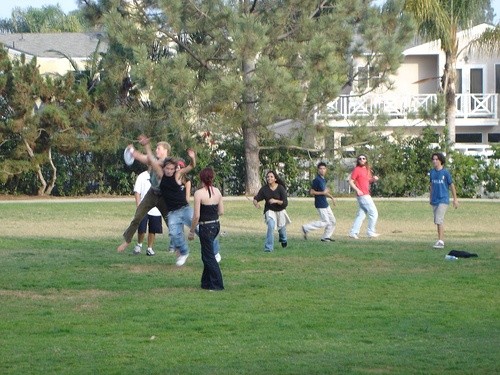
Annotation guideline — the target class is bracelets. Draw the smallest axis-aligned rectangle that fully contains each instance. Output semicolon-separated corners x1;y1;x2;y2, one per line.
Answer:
130;147;134;153
190;230;195;233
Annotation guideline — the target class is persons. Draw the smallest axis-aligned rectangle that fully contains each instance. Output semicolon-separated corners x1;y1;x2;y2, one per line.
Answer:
188;167;225;292
117;141;186;257
138;134;222;266
428;152;459;249
134;158;163;255
168;157;191;254
253;171;291;252
348;153;380;239
302;162;336;242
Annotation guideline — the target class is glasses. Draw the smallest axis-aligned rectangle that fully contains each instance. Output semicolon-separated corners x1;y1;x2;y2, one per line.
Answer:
358;158;365;160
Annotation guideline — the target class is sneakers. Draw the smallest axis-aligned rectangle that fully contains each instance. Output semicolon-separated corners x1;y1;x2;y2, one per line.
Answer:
176;253;189;266
215;253;221;263
281;242;287;247
303;226;307;240
133;243;142;252
349;234;358;239
433;241;444;248
146;247;155;255
320;238;335;242
368;232;379;237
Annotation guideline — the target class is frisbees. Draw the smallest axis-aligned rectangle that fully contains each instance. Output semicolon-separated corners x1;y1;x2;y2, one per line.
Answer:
124;145;135;165
